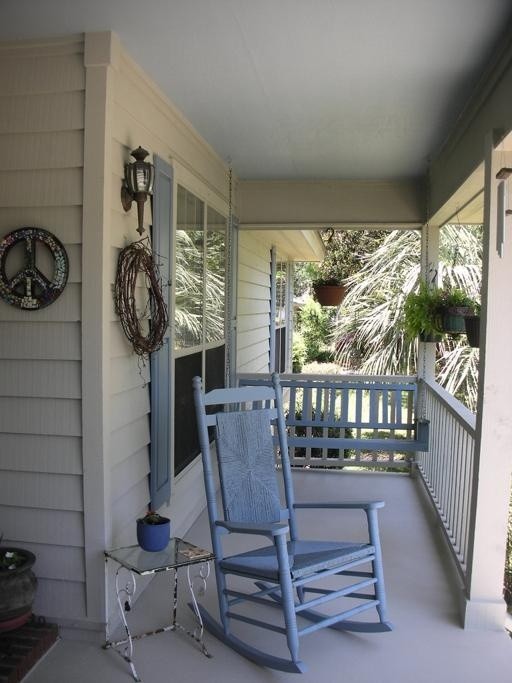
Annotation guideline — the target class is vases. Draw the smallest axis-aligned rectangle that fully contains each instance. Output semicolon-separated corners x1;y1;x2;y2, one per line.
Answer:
0;546;40;633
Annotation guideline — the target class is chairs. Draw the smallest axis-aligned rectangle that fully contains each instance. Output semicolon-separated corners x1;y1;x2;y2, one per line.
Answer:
190;370;393;676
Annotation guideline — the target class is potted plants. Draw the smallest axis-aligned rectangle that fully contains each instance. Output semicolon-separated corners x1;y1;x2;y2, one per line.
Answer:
311;270;347;307
402;286;481;350
137;511;171;552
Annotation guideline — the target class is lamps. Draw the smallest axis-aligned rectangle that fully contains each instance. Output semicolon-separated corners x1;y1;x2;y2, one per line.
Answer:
121;146;156;236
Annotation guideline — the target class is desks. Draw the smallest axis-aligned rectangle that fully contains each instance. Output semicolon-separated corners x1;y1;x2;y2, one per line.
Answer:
103;537;224;683
